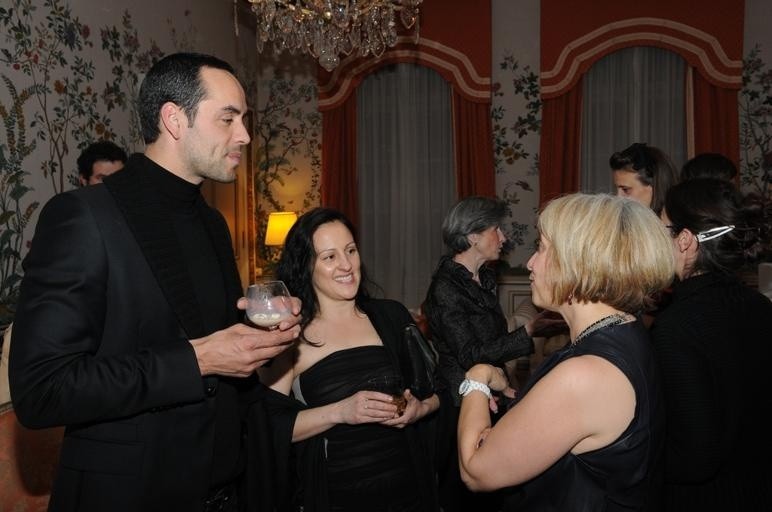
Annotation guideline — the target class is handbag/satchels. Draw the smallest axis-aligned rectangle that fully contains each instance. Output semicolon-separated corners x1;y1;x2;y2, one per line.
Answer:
400;325;437;397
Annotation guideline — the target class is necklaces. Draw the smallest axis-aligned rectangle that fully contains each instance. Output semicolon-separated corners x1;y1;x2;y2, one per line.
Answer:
570;314;623;349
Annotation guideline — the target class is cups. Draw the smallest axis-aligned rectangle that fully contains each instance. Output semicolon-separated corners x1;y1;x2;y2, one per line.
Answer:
368;375;406;414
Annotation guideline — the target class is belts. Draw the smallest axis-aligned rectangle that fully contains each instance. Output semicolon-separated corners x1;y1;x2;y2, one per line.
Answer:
204;483;238;512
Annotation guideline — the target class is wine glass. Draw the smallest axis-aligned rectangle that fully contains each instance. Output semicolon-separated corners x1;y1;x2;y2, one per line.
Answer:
243;282;294;345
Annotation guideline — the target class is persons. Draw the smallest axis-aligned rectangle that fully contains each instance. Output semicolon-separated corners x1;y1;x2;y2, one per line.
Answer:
8;50;304;512
260;206;451;512
420;195;541;512
77;140;128;187
651;177;772;512
680;152;738;184
609;142;678;218
455;193;676;512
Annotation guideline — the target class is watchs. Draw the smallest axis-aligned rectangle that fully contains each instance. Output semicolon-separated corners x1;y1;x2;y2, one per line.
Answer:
458;377;492;399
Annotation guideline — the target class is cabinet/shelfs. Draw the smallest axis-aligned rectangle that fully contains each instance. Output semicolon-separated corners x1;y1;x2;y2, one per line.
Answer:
493;273;758;405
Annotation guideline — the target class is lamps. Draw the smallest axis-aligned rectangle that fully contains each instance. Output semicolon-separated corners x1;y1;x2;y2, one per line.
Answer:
247;0;423;72
263;212;299;246
